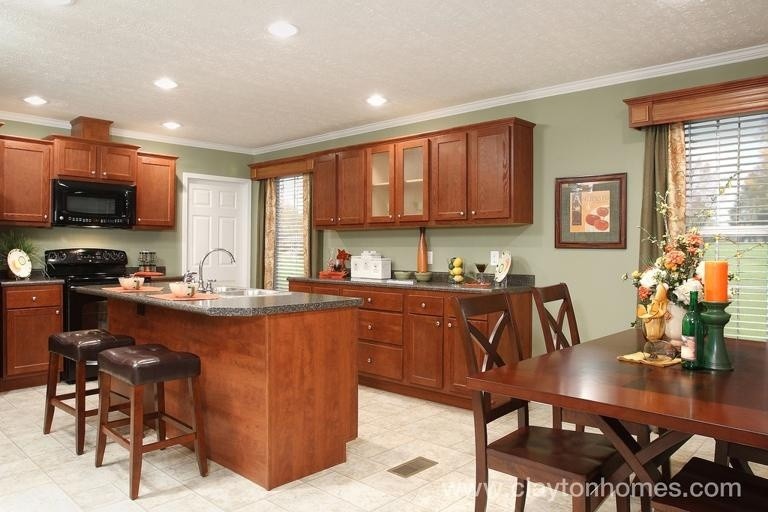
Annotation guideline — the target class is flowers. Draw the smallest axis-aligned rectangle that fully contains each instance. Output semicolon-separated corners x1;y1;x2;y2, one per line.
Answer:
621;171;768;305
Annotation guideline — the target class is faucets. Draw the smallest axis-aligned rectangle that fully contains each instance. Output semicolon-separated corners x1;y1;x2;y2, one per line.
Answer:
199;247;235;293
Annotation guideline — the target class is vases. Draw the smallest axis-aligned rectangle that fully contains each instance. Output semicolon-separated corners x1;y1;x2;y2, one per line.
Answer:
663;302;686;346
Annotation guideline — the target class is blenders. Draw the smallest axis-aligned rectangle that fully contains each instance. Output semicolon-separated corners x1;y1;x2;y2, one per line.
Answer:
137;251;157;272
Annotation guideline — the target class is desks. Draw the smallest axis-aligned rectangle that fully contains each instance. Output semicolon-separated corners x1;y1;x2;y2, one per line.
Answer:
465;328;767;512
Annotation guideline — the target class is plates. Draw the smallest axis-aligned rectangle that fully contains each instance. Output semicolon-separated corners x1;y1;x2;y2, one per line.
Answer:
494;253;512;283
7;248;32;278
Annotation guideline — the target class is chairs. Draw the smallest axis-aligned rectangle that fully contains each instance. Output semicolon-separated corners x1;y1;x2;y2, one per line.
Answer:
651;456;768;511
532;283;671;498
454;291;633;512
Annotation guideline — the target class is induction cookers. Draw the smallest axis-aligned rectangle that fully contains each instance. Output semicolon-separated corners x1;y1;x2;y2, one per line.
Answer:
44;248;130;285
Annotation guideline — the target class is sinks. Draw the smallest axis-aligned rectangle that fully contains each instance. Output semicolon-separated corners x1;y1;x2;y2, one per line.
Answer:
219;287;281;298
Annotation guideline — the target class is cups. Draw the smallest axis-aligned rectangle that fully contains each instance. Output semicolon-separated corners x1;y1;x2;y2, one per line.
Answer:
333;259;344;272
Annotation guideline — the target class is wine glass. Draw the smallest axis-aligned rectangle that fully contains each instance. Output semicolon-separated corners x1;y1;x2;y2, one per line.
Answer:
642;317;667;362
474;263;489;284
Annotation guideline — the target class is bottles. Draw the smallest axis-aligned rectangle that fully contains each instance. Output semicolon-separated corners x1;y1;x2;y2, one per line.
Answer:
680;290;705;371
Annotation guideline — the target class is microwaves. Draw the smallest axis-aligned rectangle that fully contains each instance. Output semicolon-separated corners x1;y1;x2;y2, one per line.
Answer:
52;179;136;229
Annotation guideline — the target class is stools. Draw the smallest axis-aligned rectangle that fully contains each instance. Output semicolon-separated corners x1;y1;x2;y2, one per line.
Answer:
43;328;135;454
96;345;209;500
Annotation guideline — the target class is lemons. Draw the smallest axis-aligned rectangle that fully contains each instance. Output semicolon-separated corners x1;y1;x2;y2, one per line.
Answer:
449;257;463;283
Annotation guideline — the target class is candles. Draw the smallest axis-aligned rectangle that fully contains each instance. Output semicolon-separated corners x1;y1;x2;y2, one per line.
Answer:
698;261;733;301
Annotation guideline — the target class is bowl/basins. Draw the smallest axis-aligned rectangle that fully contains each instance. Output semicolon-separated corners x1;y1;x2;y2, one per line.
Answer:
168;282;200;298
414;271;433;282
118;276;145;290
394;271;412;280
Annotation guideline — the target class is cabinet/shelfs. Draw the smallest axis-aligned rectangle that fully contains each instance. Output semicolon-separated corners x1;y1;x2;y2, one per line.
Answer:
312;117;536;231
52;135;139;182
137;152;179;229
1;284;64;392
0;136;54;227
290;281;532;412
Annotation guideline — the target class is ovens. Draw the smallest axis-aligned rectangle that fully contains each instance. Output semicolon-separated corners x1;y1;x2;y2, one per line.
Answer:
61;287;107;385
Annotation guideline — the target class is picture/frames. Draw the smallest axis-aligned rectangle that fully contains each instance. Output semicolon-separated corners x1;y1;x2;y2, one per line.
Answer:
555;173;626;249
